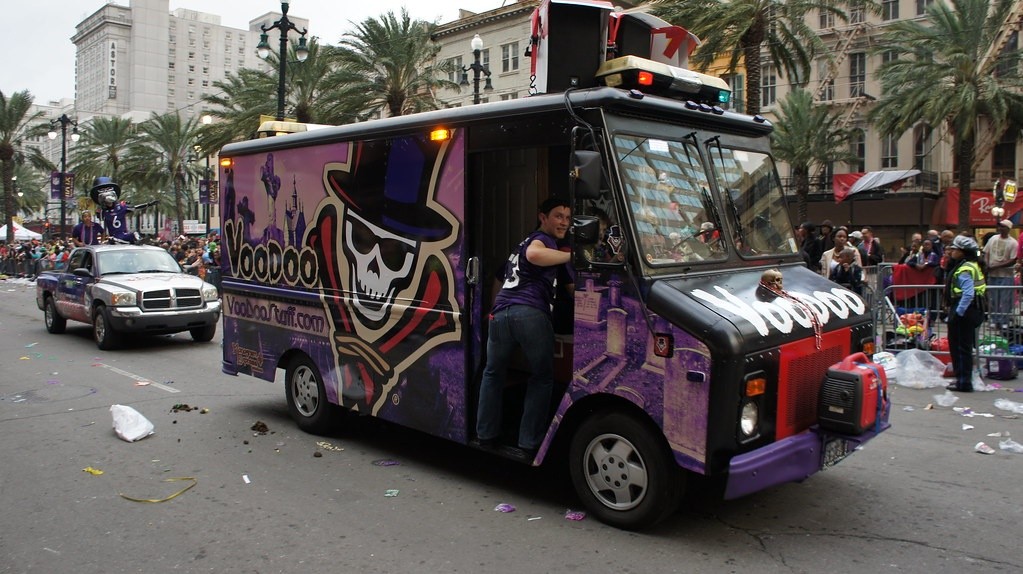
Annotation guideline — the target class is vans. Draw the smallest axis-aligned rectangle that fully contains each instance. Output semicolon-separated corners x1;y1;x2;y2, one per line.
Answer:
217;55;893;531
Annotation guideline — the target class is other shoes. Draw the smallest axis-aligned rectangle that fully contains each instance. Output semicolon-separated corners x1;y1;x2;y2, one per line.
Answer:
944;316;949;322
950;380;957;385
989;323;996;328
1002;323;1008;329
478;438;498;447
929;320;934;326
519;448;536;458
946;384;972;391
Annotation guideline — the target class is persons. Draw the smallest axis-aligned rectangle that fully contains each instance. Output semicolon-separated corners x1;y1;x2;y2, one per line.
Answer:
71;211;106;248
982;218;1019;324
0;231;222;293
477;195;611;456
818;225;863;279
946;234;987;392
791;220;1023;327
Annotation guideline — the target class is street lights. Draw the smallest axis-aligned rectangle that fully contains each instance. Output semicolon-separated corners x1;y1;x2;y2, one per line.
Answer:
458;33;494;105
46;113;81;240
256;0;310;121
11;175;24;216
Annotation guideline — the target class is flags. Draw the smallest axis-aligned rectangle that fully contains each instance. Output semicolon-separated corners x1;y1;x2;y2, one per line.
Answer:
833;168;922;205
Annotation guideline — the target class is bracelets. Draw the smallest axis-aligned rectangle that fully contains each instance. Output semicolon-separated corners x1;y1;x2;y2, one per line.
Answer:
570;250;574;262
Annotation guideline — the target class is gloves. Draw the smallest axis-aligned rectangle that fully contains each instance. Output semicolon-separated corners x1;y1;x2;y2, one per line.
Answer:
948;312;961;327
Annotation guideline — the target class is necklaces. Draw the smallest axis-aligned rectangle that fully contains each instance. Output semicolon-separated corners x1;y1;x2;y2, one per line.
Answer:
81;221;92;245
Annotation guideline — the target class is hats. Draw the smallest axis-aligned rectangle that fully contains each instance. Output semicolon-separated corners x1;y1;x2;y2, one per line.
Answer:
535;197;570;232
848;231;864;239
1000;219;1013;229
815;220;833;232
950;236;979;253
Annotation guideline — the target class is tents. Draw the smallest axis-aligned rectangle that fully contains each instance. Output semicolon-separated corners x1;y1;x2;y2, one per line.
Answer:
0;219;44;240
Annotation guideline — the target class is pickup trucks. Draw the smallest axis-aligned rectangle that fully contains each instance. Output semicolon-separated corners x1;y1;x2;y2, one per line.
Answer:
36;242;222;349
